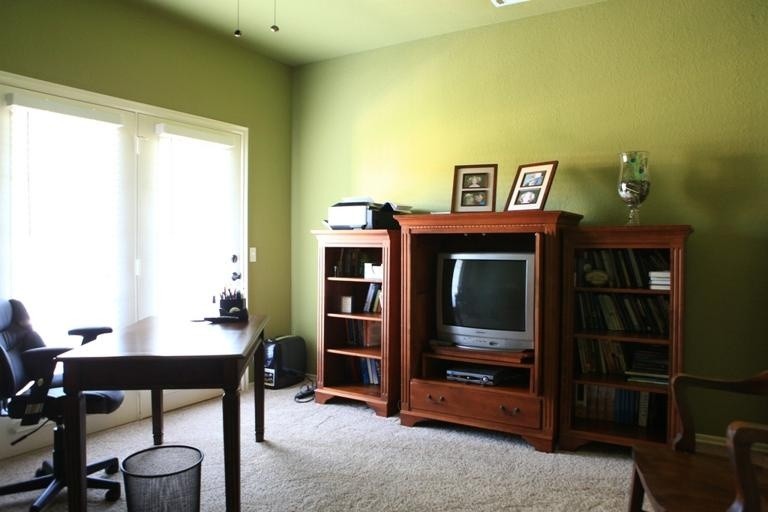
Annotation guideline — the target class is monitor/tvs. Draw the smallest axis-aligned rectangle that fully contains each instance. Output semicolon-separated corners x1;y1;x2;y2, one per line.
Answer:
436;252;535;353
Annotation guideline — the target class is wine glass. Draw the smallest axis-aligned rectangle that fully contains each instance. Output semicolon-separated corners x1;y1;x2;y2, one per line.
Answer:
615;151;650;224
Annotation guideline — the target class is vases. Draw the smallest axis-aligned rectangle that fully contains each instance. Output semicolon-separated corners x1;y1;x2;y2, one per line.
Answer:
612;148;657;227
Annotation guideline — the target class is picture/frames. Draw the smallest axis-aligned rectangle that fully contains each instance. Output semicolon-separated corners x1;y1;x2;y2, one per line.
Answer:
450;163;498;213
503;159;559;211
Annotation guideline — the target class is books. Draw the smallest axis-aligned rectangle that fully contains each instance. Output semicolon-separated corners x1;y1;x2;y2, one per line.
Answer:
330;248;383;384
571;244;672;431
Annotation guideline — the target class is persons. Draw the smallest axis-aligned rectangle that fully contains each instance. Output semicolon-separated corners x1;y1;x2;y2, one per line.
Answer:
463;174;487;206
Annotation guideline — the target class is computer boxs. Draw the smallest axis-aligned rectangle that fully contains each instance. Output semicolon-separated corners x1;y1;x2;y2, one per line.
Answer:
263;335;307;389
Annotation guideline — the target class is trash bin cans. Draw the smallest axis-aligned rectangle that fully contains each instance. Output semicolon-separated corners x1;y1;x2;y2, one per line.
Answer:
120;445;204;512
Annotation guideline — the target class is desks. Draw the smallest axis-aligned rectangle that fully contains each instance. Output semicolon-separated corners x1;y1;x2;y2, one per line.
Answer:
49;306;278;512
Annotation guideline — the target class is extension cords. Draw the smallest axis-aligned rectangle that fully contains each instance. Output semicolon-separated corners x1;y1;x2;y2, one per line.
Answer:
296;386;318;399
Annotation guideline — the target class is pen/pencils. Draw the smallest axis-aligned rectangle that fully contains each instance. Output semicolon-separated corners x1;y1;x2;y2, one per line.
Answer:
220;287;244;300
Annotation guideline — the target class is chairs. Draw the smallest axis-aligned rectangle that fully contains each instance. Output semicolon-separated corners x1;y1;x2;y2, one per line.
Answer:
0;291;124;512
619;361;768;511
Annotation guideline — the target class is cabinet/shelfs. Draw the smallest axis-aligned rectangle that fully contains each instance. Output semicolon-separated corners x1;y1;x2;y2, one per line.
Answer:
392;210;583;454
562;224;695;457
309;225;403;418
